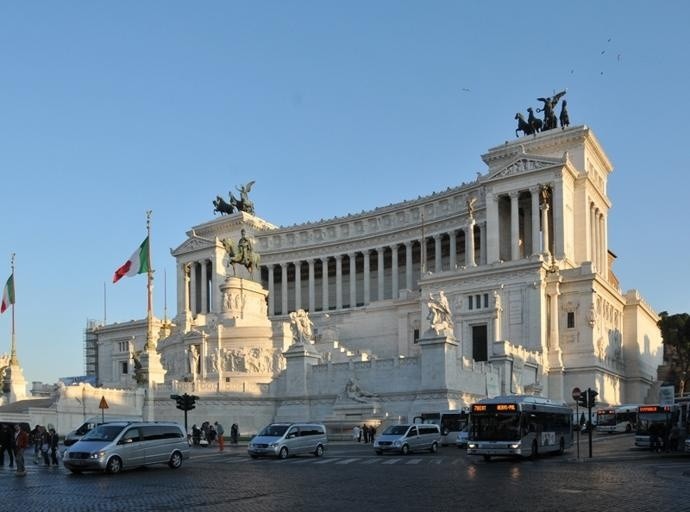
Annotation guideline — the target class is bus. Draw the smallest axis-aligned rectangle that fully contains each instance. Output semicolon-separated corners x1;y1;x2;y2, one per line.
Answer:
413;393;690;465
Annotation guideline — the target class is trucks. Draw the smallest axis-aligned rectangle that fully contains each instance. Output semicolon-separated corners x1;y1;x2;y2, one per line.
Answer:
62;414;144;446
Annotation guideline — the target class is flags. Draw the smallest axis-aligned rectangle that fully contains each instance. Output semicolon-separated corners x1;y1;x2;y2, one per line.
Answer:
0;274;15;314
112;236;150;283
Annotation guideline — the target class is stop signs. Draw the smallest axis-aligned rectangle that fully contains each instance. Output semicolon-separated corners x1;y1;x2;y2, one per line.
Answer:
571;387;582;401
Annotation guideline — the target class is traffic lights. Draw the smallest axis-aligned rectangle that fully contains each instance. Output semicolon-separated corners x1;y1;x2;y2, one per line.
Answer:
589;388;598;407
175;395;185;411
578;390;588;408
186;395;196;411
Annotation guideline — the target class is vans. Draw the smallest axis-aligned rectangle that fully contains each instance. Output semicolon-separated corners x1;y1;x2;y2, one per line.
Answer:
58;419;192;478
245;421;330;461
0;421;34;448
372;422;443;456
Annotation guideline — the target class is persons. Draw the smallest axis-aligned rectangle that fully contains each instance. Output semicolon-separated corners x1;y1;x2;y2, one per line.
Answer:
436;290;451;323
187;420;240;452
11;422;27;476
354;424;375;444
238;227;252;266
0;422;14;468
647;418;688;454
288;309;313;346
29;423;59;468
